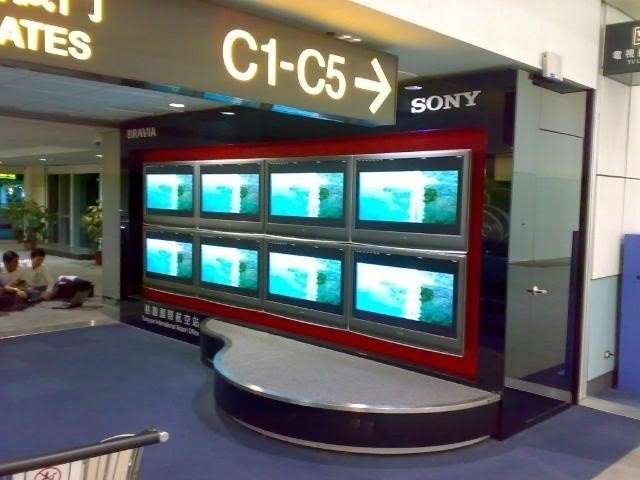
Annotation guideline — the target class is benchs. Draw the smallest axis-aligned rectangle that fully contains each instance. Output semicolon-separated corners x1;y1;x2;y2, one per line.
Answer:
200;315;500;457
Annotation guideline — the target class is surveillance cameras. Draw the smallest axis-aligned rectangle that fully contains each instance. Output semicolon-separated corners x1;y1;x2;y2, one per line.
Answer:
94;137;101;146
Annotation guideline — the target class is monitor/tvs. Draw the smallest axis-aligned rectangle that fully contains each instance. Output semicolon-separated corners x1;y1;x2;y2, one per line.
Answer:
264;234;351;329
201;159;263;231
354;150;473;253
264;154;355;242
198;231;264;310
352;246;467;357
140;161;197;227
144;228;198;294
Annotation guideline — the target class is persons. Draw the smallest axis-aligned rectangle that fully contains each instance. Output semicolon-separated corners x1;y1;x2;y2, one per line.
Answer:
19;248;53;302
1;250;35;312
